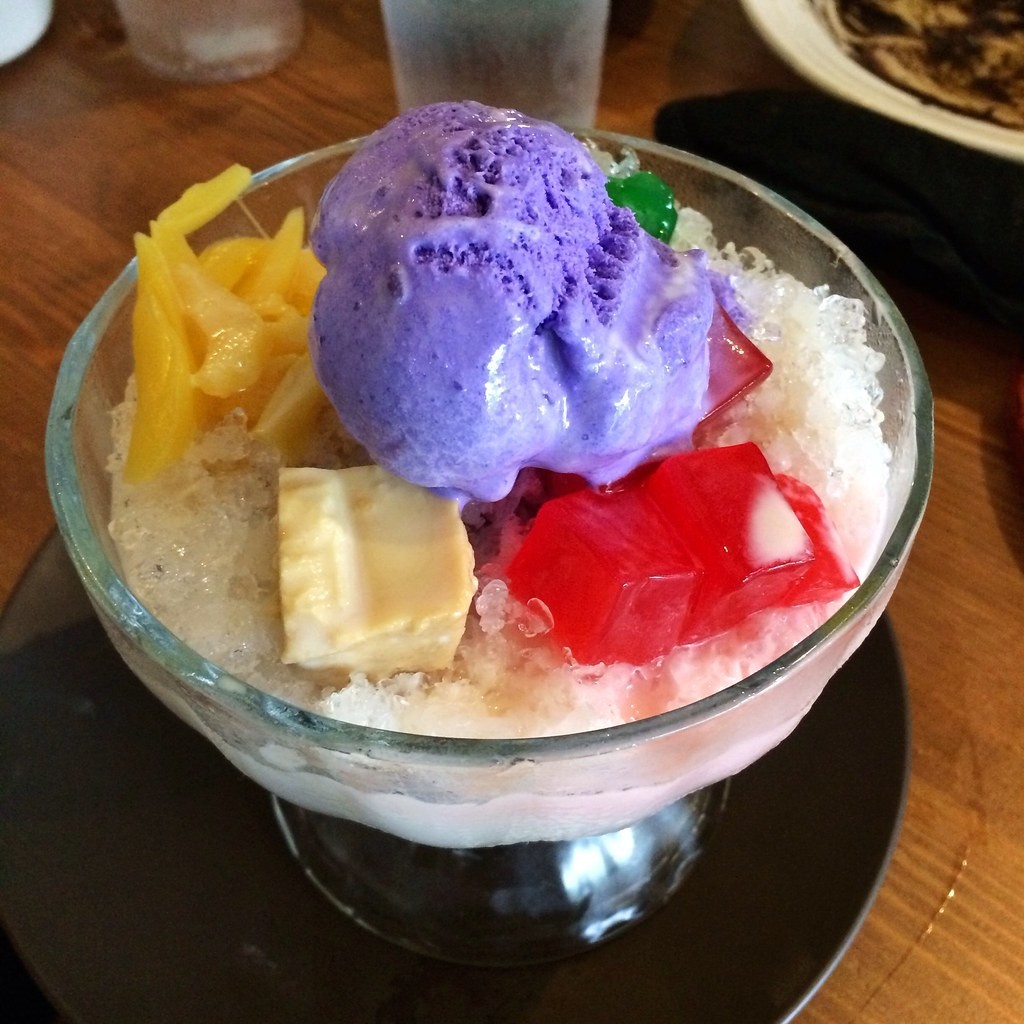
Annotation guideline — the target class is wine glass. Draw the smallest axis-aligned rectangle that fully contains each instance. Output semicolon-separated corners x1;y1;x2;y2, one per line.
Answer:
42;124;937;969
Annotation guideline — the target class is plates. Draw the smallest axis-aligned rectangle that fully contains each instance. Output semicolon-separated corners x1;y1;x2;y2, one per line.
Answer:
741;0;1024;164
0;524;910;1024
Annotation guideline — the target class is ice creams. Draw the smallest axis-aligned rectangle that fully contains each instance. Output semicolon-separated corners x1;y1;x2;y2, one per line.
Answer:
301;96;761;508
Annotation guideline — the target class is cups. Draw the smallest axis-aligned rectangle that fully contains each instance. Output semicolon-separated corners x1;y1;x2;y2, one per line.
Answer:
379;0;610;126
0;0;55;65
114;0;303;84
